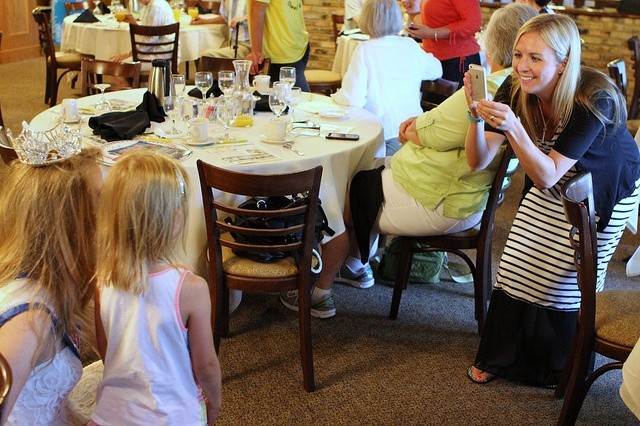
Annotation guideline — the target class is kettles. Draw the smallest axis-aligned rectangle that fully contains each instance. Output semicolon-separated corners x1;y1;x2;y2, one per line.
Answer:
147;58;176;113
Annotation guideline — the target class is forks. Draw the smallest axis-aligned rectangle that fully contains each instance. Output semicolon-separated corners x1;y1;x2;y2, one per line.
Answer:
284;144;305;157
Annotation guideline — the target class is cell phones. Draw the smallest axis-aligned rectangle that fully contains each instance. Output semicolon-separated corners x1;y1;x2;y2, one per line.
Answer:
409;27;417;30
469;64;488;102
326;133;359;140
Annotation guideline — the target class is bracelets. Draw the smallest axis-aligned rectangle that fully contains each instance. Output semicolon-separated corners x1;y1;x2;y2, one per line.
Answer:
436;27;438;41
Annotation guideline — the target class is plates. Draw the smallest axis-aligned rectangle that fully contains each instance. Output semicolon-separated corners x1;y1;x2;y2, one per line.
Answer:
93;139;190;167
260;134;295;143
300;100;349;118
185;138;215;146
350;33;371;41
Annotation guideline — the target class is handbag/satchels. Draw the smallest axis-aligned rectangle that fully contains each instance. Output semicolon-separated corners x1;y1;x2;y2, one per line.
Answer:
224;192;335;274
378;237;474;283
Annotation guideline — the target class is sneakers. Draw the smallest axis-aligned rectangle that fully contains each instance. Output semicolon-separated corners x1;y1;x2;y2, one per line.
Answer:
333;263;375;288
280;290;336;318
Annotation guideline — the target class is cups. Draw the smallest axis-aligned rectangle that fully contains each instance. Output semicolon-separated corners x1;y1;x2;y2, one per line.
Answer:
189;117;210;143
264;119;288;141
253;75;269;95
62;98;80;119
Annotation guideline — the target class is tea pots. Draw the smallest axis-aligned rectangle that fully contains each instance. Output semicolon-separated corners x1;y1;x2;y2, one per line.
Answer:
233;58;254;121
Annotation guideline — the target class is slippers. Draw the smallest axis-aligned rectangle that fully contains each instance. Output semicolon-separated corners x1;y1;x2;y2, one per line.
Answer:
467;365;496;384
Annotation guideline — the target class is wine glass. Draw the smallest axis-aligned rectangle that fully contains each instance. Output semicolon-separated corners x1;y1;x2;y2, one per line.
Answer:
188;7;198;24
94;84;111;117
195;71;235;143
172;8;181;23
115;5;124;25
269;67;301;120
166;74;194;136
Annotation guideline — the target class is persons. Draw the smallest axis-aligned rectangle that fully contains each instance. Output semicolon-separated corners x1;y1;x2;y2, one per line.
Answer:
463;12;635;394
85;152;222;426
338;0;368;38
405;0;483;84
51;0;85;52
330;0;444;153
246;0;312;93
191;0;251;59
108;0;177;74
2;148;100;426
278;4;538;318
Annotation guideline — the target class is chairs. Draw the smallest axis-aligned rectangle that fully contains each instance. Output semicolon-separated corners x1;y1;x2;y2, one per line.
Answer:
558;170;640;425
31;7;103;107
420;78;460;112
67;2;89;16
0;108;19;165
626;35;640;120
128;22;180;83
80;57;140;98
303;13;345;97
198;1;219;14
197;159;324;392
199;55;271;85
607;57;640;137
381;140;512;336
